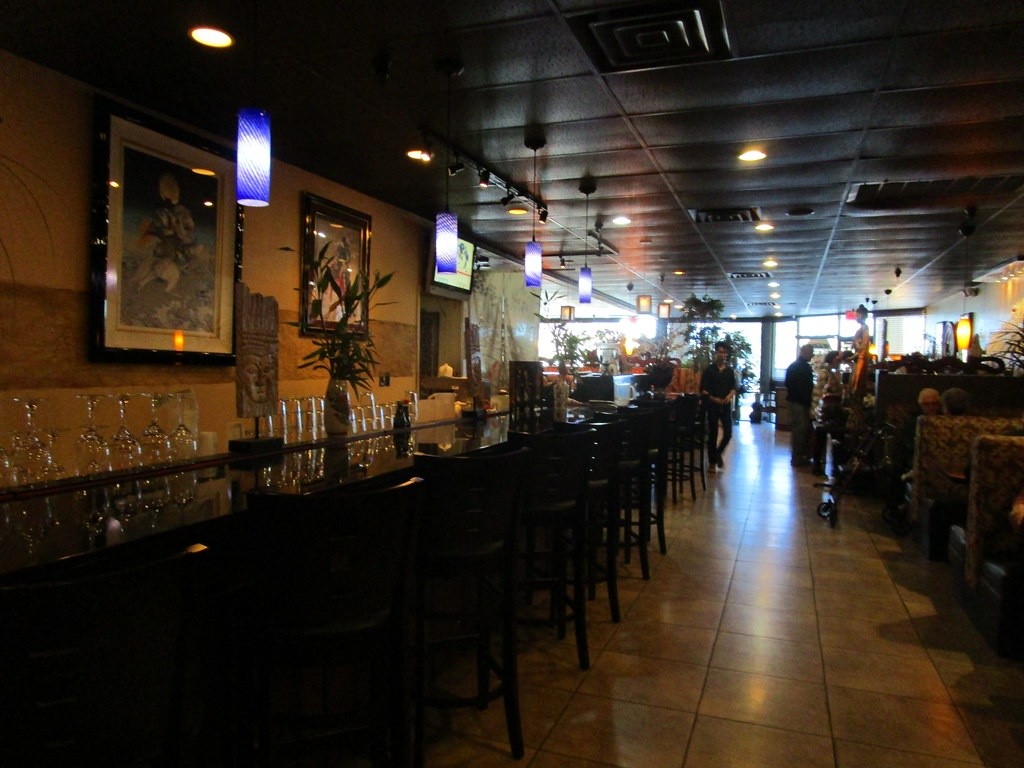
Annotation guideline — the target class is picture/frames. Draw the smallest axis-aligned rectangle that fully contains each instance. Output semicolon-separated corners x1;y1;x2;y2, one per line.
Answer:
84;92;238;364
297;189;368;338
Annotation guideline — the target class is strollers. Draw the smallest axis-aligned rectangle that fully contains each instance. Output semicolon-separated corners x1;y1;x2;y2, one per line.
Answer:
811;392;908;524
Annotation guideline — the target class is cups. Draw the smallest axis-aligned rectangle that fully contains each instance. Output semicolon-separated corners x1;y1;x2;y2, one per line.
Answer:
224;391;464;448
242;430;417;494
199;432;218;455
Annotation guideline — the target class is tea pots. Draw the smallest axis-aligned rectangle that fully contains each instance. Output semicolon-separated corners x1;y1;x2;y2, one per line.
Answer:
439;363;453;377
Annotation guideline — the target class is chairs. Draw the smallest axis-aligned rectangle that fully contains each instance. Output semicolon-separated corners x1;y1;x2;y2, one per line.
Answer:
240;390;712;768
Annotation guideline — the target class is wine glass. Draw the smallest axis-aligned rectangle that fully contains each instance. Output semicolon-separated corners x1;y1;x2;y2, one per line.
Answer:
0;467;198;567
0;394;80;491
166;390;198;466
107;393;142;476
75;394;112;480
140;392;176;471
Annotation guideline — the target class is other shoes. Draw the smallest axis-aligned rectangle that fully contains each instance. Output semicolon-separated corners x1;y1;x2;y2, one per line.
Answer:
716;449;724;468
708;463;716;473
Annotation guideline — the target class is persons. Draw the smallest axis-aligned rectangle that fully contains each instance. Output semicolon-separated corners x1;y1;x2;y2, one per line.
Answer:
894;350;965;374
889;388;972;517
964;422;1024;477
844;306;870;427
785;344;814;466
1009;489;1024;533
811;352;842;477
701;342;736;472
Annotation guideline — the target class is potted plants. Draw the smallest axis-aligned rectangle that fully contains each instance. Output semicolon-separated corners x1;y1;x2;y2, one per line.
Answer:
528;286;592;422
289;239;396;433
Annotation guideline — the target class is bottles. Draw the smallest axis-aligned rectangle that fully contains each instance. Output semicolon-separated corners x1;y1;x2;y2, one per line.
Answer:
393;434;403;458
600;362;614;401
554;373;569;422
167;378;200;445
402;432;410;457
401;400;411;427
393;401;409;428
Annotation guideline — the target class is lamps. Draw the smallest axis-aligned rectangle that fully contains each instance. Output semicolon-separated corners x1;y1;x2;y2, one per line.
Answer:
499;183;514;206
476;164;488;188
956;280;971;351
235;0;272;207
524;126;547;285
436;63;464;272
578;172;597;303
536;204;548;224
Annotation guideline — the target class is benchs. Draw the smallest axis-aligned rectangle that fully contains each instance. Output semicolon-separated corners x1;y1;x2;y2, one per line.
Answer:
880;399;1023;593
